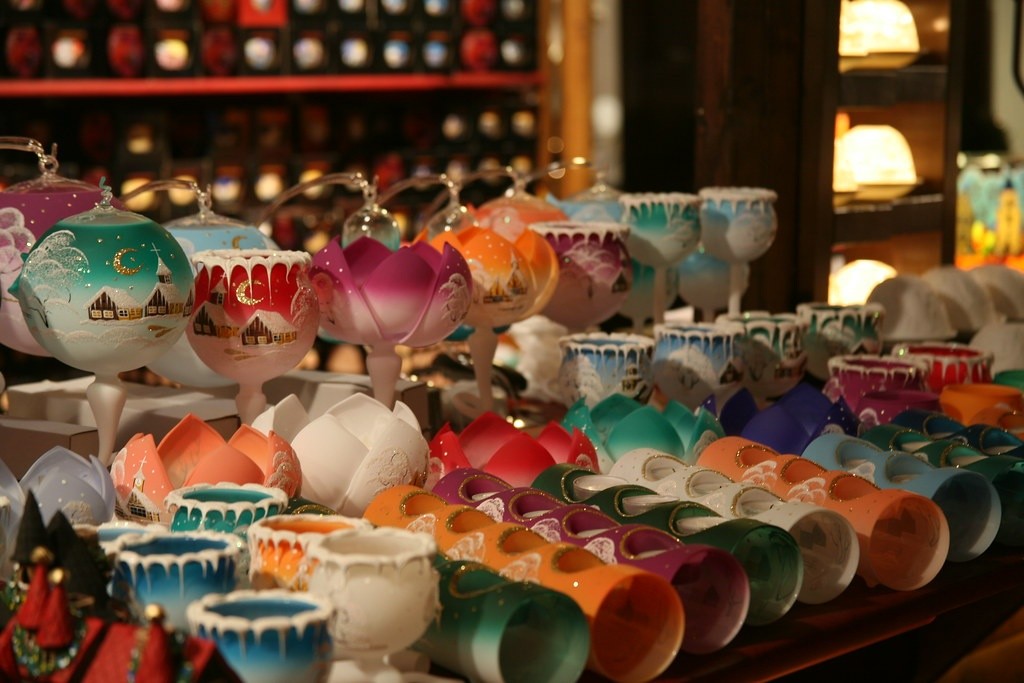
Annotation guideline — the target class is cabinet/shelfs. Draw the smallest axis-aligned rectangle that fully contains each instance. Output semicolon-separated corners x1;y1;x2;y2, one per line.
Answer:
2;1;622;220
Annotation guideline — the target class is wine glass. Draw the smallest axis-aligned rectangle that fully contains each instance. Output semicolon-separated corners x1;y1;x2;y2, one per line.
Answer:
522;189;995;418
194;248;318;428
82;483;440;682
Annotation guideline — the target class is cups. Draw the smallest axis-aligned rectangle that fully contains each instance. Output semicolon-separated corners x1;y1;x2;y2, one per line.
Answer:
360;368;1024;681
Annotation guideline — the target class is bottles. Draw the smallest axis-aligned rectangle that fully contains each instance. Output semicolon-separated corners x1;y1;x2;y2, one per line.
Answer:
5;104;537;243
2;2;523;77
